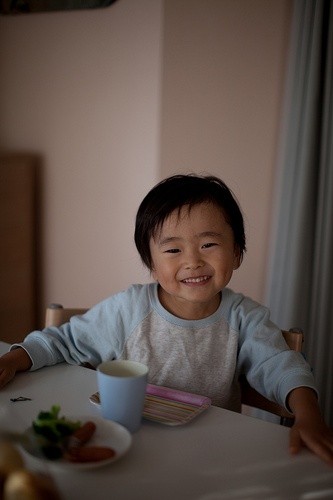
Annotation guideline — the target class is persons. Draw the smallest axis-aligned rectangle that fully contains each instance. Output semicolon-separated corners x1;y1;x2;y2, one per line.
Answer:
0;173;333;470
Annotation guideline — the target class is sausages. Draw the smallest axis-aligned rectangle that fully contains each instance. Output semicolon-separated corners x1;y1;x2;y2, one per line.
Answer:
71;422;96;444
67;446;117;460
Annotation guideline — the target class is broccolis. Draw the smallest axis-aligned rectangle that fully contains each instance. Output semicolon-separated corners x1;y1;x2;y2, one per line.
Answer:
24;405;79;460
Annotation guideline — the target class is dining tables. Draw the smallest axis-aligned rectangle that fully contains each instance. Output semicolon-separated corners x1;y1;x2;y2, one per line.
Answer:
0;341;332;500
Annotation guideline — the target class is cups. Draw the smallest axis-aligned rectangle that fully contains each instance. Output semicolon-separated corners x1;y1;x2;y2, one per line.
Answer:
97;358;148;435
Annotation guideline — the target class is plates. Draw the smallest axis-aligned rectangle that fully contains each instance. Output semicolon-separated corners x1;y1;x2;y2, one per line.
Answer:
89;382;212;427
19;414;135;472
